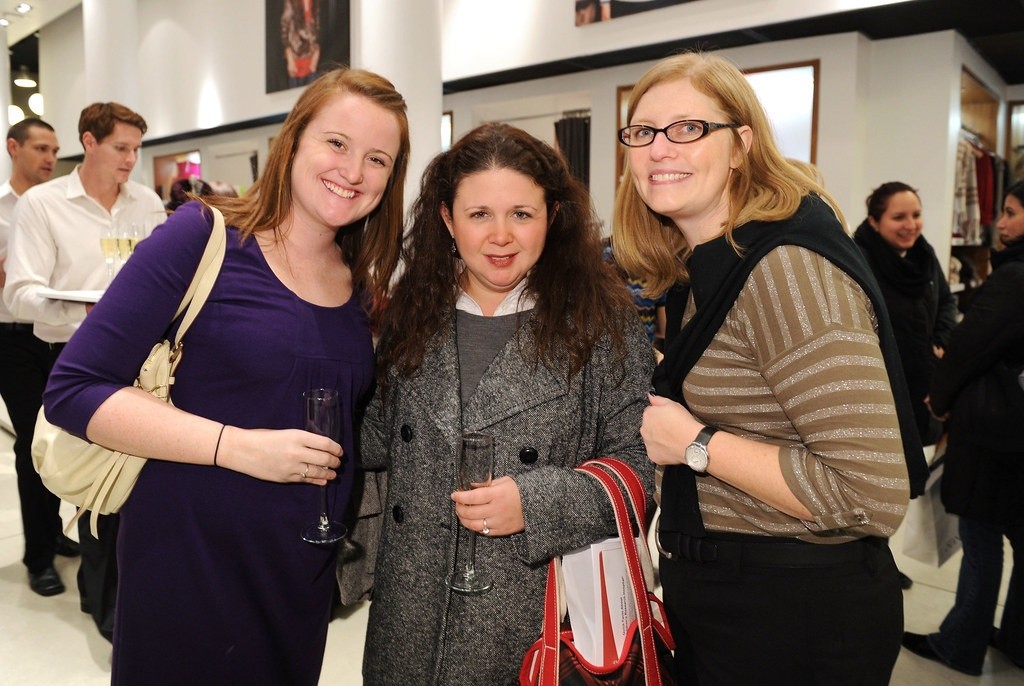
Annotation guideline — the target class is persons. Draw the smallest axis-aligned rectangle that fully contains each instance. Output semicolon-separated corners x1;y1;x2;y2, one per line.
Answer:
1;102;238;646
44;67;411;686
848;179;1024;676
361;120;656;686
604;244;668;349
612;51;931;686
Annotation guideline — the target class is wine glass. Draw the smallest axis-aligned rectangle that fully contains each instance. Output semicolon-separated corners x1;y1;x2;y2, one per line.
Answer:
444;431;493;592
302;386;350;544
96;220;145;297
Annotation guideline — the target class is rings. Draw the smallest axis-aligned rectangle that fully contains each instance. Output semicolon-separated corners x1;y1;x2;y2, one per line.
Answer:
481;519;489;533
303;462;310;478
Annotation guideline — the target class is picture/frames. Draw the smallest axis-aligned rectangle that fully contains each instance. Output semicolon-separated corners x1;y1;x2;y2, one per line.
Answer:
615;59;821;198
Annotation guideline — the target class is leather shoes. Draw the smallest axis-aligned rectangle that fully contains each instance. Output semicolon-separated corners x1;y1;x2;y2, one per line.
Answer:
31;568;64;596
59;536;80;557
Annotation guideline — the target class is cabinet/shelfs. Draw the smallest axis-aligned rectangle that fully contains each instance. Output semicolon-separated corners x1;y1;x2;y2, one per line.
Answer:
946;71;1024;297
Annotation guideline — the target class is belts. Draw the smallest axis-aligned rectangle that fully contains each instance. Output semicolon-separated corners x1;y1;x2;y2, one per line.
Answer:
0;323;33;330
655;516;888;564
32;334;66;351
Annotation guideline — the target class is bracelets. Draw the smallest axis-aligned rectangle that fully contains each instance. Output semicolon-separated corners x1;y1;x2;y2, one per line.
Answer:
214;425;226;466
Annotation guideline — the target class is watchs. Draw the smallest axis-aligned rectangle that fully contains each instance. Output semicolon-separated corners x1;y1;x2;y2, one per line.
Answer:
686;424;721;476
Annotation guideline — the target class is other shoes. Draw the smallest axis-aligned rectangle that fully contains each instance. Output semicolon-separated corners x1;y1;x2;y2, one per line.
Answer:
902;631;940;661
989;626;1003;650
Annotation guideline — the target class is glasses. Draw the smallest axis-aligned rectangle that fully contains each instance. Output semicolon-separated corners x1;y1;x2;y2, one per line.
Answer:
618;120;739;147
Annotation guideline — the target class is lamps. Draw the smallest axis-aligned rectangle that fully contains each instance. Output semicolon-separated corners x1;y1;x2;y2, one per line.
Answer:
15;64;36;88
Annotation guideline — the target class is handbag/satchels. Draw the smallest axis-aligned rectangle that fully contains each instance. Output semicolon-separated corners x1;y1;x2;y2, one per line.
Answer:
900;422;963;568
519;458;678;686
31;205;226;540
